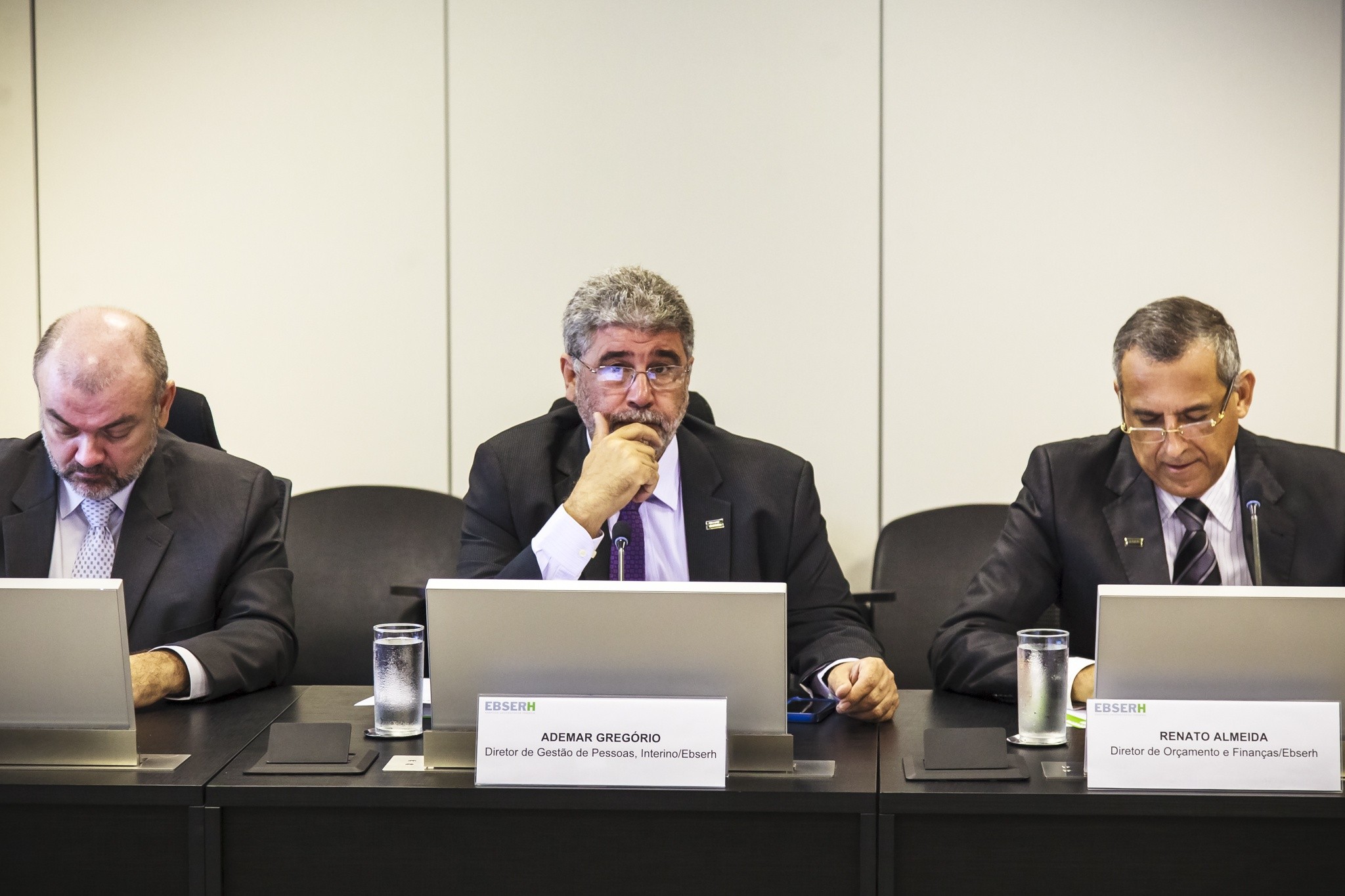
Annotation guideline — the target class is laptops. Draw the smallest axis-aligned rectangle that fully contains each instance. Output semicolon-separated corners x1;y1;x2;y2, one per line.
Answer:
0;578;135;731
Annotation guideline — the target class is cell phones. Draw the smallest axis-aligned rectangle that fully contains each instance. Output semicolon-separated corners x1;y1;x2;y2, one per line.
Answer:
787;697;836;724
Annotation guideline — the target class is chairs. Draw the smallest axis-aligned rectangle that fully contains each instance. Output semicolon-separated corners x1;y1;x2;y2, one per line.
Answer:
283;484;463;686
872;503;1018;688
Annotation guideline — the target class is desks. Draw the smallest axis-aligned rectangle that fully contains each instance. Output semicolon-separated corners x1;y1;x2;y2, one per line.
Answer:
0;690;1345;895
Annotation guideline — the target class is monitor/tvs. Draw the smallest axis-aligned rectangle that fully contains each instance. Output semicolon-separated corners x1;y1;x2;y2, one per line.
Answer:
425;578;788;737
1094;585;1345;740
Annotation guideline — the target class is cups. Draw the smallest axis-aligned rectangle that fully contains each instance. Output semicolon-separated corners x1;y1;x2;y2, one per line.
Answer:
373;623;424;737
1017;628;1069;743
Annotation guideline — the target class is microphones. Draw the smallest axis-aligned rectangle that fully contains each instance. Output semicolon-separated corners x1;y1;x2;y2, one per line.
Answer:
1241;479;1263;586
612;521;631;580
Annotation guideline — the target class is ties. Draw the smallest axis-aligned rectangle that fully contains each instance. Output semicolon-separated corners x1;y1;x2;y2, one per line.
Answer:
1174;498;1222;586
609;500;645;582
70;497;117;578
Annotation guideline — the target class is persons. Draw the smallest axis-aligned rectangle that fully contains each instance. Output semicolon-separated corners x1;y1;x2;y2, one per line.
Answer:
0;307;298;710
457;268;901;723
926;296;1345;710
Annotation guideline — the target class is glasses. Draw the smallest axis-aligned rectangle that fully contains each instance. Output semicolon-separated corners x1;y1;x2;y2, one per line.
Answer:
569;351;690;392
1120;372;1236;443
